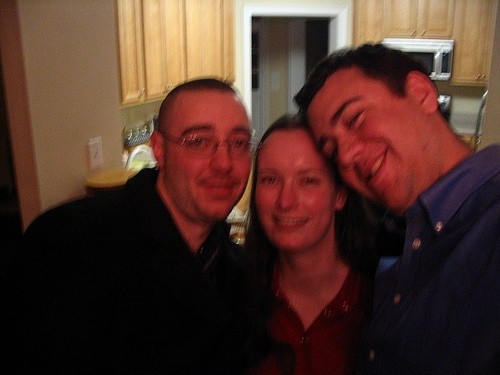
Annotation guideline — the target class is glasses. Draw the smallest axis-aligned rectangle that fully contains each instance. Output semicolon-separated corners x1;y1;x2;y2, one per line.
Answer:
168;129;262;162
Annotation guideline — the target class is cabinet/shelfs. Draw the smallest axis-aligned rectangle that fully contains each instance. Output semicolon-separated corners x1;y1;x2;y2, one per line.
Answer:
352;0;498;88
116;0;236;106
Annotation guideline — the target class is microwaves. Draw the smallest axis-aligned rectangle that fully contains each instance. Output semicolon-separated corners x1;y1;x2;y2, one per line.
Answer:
380;38;455;82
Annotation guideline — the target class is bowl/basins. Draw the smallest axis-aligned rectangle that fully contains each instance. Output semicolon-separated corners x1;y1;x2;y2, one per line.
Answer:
126;145;157;172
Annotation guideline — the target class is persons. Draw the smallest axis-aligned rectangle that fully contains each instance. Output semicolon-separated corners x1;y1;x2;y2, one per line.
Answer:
0;78;263;375
243;114;366;375
292;42;500;375
